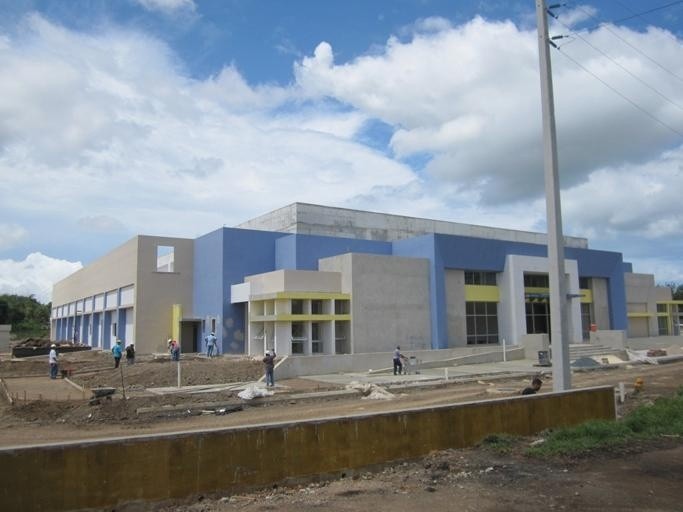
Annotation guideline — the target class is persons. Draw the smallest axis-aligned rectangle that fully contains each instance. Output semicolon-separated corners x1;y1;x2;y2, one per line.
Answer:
170;339;180;361
519;377;542;396
390;345;405;376
261;348;276;388
166;339;172;353
111;339;123;369
204;332;217;357
46;342;60;380
124;343;135;366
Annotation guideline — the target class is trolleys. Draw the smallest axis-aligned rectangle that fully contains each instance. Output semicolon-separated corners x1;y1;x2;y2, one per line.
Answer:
403;357;423;375
89;387;116;401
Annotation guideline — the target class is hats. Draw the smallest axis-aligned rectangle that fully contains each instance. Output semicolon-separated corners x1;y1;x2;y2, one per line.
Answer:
117;340;122;343
51;344;57;348
168;339;171;343
265;350;270;355
